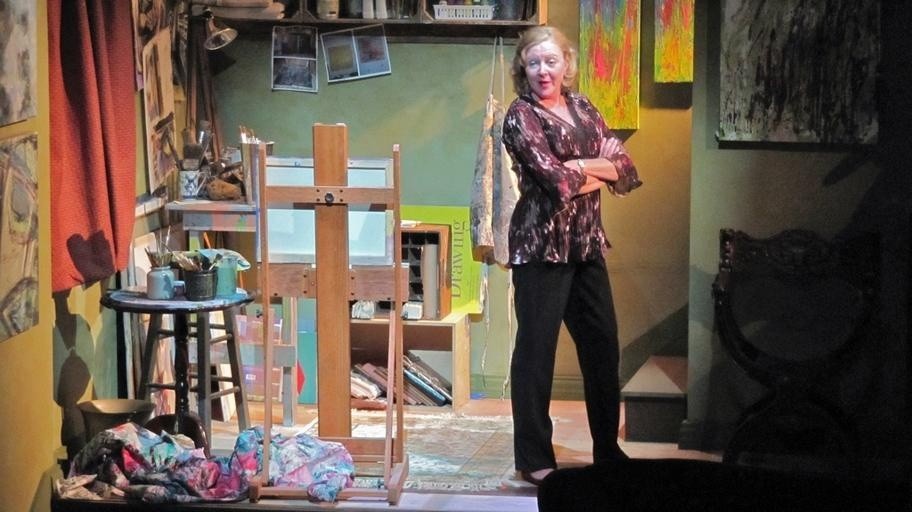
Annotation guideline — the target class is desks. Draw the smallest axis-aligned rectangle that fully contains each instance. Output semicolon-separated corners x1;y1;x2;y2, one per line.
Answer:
167;198;259;264
103;287;253;456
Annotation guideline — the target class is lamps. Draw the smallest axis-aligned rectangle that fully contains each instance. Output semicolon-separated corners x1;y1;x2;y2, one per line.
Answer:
200;5;240;54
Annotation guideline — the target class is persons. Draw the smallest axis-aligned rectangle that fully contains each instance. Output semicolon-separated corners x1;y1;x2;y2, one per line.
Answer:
497;25;643;483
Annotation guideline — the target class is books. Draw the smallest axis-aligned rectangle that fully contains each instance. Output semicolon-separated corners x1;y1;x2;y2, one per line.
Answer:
351;353;452;407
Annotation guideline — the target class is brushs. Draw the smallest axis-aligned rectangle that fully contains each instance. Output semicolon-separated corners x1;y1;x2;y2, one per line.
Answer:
183;144;202;170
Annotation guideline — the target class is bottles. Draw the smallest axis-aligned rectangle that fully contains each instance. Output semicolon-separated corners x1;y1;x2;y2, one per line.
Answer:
146;265;177;300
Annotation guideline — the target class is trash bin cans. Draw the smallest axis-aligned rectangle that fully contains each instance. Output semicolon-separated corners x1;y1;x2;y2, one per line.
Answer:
82;400;157;449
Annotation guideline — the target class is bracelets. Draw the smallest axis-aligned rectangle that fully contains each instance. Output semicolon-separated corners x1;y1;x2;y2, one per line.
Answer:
577;158;587;171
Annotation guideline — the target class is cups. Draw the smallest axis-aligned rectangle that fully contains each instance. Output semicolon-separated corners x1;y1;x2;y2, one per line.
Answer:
181;267;218;302
178;170;207;201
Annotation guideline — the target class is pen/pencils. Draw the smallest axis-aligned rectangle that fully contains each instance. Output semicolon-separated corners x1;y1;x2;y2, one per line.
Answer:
144;226;173;267
176;251;223;272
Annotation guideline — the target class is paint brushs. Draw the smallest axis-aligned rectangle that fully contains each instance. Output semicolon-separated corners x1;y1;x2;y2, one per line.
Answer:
181;120;214;169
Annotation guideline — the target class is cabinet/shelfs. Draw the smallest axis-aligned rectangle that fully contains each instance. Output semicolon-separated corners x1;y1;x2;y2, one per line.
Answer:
343;315;471;416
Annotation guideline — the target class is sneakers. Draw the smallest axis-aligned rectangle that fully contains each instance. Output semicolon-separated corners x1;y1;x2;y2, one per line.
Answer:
519;466;558;485
